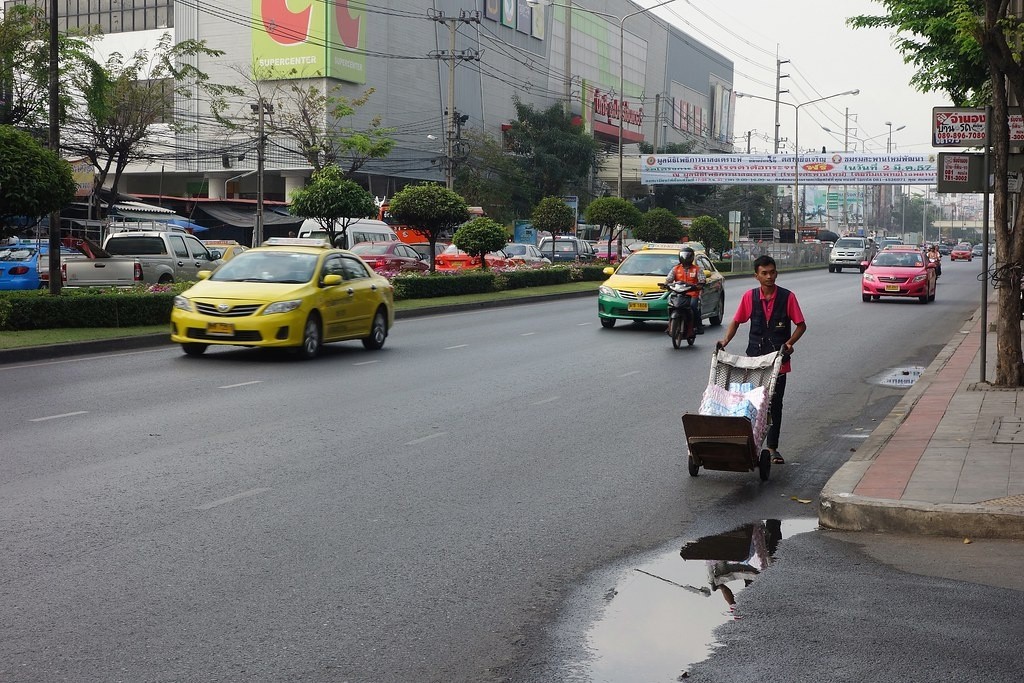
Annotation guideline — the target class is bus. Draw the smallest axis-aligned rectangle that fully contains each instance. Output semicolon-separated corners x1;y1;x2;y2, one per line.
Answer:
378;205;485;243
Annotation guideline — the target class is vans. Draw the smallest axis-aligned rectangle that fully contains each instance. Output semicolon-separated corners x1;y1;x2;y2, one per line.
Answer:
297;216;400;251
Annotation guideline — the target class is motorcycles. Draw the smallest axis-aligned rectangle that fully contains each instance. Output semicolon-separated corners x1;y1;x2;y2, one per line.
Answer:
657;280;700;349
928;256;943;281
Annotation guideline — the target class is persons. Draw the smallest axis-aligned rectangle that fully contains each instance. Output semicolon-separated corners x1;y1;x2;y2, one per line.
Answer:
716;256;806;464
927;245;943;275
662;248;706;333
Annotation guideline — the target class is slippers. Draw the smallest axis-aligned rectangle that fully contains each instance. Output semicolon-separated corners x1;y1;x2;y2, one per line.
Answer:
770;451;784;464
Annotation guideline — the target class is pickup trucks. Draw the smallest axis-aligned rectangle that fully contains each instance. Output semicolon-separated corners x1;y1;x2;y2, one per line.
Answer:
36;215;190;287
61;230;224;288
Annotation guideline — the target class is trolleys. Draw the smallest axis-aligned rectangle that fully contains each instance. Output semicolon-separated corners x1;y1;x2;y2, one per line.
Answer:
681;341;788;481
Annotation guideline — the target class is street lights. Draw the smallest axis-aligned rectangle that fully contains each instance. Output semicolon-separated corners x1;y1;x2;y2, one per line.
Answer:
736;89;907;241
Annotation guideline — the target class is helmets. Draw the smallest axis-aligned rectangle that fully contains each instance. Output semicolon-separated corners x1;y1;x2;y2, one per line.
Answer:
679;248;694;266
929;245;934;250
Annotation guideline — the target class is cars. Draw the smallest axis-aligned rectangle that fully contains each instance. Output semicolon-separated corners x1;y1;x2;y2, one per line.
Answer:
409;243;450;260
860;250;936;305
199;240;251;264
350;242;429;272
828;235;876;274
170;238;395;360
598;242;725;335
501;236;638;270
435;244;518;269
0;243;81;290
941;241;996;262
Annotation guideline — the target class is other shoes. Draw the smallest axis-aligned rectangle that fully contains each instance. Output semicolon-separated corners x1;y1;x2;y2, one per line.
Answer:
664;329;669;332
697;322;704;334
